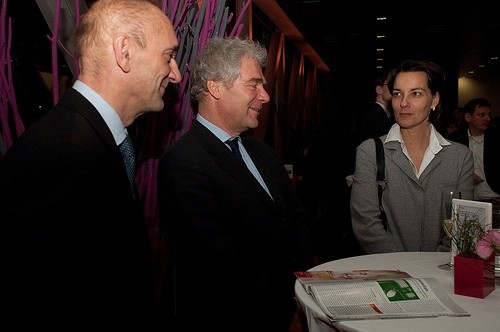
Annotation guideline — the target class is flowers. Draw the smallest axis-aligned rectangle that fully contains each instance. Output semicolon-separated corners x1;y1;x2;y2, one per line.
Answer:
439;201;500;259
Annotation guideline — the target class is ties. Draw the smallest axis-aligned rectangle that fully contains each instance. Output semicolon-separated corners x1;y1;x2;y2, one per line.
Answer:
118;133;135;202
226;137;253;177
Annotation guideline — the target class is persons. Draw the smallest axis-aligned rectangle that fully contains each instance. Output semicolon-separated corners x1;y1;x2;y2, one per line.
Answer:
0;0;182;332
294;270;411;281
158;37;310;332
350;60;500;254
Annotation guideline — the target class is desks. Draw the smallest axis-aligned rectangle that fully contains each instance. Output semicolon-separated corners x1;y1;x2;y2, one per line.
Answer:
295;251;500;332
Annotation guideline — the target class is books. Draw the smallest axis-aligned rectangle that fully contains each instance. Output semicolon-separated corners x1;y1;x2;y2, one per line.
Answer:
294;270;471;323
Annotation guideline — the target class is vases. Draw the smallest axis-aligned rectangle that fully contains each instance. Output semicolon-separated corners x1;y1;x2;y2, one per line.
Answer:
453;255;495;299
